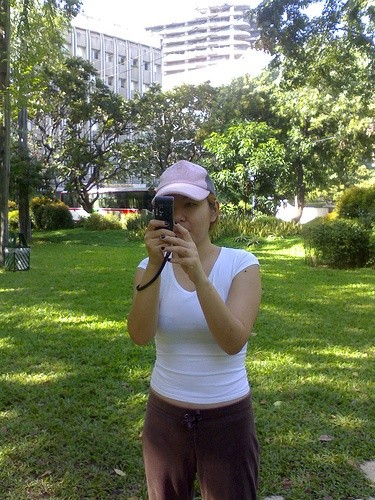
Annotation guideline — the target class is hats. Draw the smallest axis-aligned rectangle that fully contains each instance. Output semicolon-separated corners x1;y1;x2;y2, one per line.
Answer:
151;160;216;205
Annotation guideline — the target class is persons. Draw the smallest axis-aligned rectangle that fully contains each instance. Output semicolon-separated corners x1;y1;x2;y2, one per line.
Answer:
127;160;261;500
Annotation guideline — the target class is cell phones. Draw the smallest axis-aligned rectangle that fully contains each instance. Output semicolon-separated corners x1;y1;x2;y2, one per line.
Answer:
154;196;175;232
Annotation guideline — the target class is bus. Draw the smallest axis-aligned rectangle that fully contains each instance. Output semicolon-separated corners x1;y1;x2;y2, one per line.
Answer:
59;183;155;230
